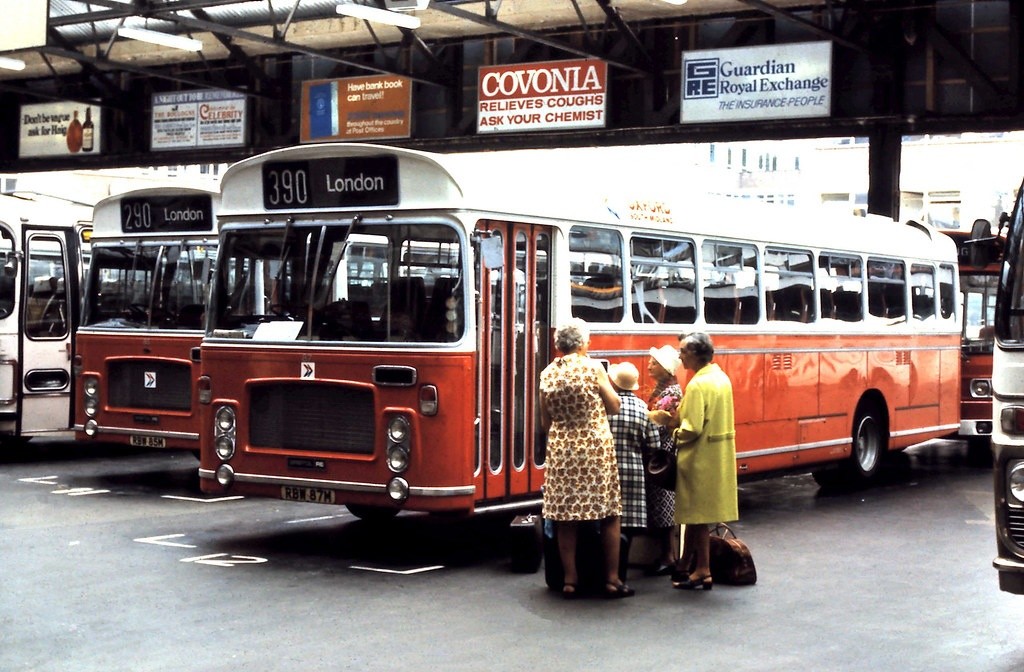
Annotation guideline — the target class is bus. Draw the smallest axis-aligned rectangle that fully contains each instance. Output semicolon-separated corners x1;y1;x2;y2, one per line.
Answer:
71;186;548;463
955;176;1024;599
0;189;94;447
195;143;963;549
937;226;1007;442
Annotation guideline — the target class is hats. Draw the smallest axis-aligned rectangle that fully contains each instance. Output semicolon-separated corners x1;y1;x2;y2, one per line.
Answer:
607;362;639;390
649;344;681;376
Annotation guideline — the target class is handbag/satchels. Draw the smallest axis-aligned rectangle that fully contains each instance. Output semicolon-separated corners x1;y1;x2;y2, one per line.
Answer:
687;522;756;586
642;430;679;491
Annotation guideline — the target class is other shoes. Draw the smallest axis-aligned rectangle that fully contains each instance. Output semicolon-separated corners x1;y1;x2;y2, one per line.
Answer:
644;559;676;577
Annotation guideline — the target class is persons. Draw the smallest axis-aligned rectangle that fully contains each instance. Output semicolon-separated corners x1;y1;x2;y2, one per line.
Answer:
538;317;740;598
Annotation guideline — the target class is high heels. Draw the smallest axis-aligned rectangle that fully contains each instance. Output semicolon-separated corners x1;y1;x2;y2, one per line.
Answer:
605;581;635;600
562;580;578;598
670;570;690;582
672;575;714;589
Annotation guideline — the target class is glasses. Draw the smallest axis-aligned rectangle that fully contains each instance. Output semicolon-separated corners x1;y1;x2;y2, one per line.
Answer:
586;340;591;346
